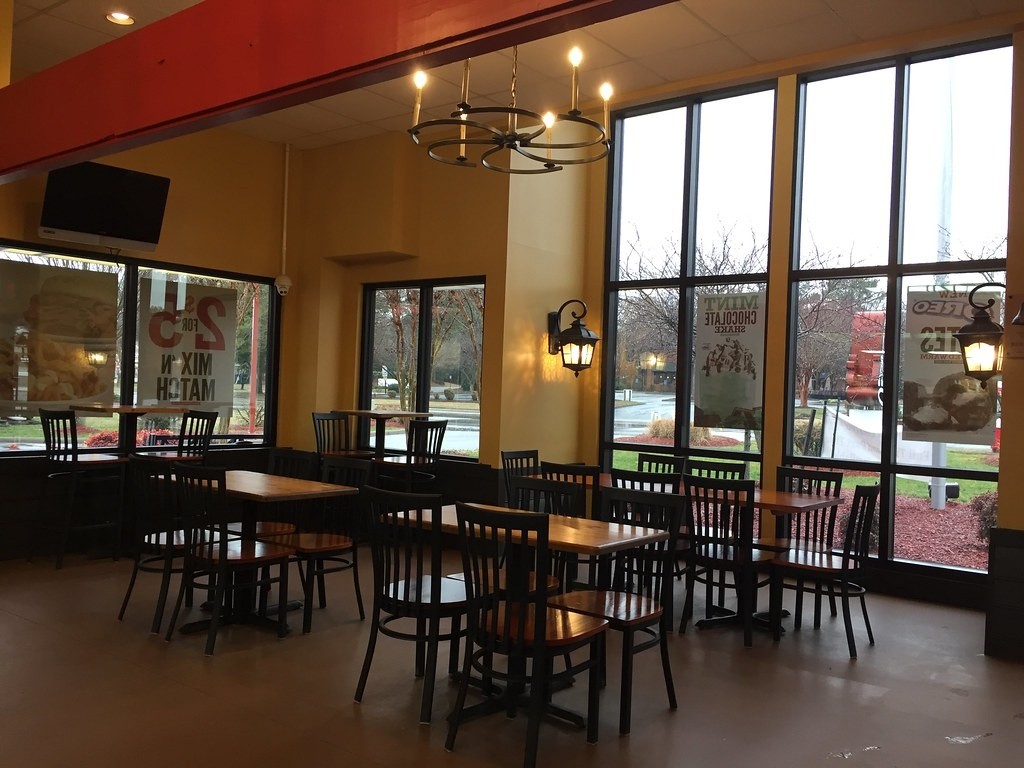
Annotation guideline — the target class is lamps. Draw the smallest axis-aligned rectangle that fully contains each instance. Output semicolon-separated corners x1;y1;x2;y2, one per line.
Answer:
949;281;1024;390
81;327;110;368
406;44;614;177
548;299;603;378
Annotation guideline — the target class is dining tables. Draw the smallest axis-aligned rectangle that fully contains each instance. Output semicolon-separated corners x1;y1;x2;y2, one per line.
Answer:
153;468;360;639
522;472;845;641
377;505;669;729
332;408;433;459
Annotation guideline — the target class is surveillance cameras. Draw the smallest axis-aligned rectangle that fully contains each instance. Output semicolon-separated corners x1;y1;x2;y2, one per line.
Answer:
274;275;293;297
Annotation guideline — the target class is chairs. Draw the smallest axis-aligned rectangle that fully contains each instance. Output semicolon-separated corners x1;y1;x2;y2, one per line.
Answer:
685;458;747;544
498;450;539;570
540;460;601;580
205;447;320;602
371;419;450;492
128;409;220;561
442;501;610;768
772;481;882;659
446;476;585;597
545;485;677;736
120;465;241;636
24;406;129;565
311;411;374;486
165;447;297;658
752;466;845;632
637;453;682;582
257;455;373;633
679;472;782;648
353;484;504;728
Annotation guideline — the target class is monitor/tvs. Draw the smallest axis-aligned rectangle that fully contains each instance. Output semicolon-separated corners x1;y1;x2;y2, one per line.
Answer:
37;161;170;253
